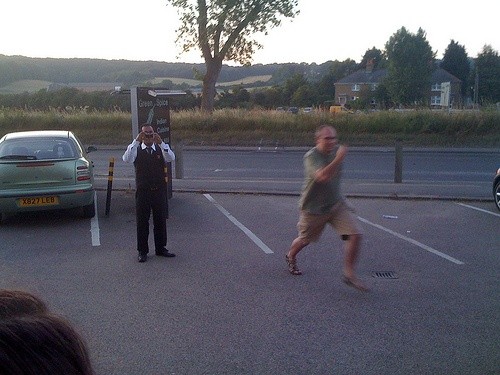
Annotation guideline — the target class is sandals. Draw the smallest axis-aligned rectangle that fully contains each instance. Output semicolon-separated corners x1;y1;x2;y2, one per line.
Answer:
341;276;365;290
285;254;302;275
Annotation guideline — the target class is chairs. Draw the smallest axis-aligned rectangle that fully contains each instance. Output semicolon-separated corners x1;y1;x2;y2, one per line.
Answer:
12;146;27;155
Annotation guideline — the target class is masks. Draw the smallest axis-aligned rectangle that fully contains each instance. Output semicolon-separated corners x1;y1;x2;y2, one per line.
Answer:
143;133;154;142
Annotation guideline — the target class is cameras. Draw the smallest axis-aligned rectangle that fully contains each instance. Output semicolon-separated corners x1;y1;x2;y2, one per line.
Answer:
145;134;153;138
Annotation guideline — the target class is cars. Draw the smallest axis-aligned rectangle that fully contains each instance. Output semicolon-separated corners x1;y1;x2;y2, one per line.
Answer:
288;107;298;114
303;108;313;113
276;107;285;112
0;130;98;225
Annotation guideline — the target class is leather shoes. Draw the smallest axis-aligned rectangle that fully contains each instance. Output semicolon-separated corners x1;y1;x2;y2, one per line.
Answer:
155;250;176;258
137;251;148;262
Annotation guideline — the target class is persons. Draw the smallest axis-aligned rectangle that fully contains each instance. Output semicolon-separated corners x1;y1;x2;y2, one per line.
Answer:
0;288;95;374
284;124;372;292
122;124;175;262
